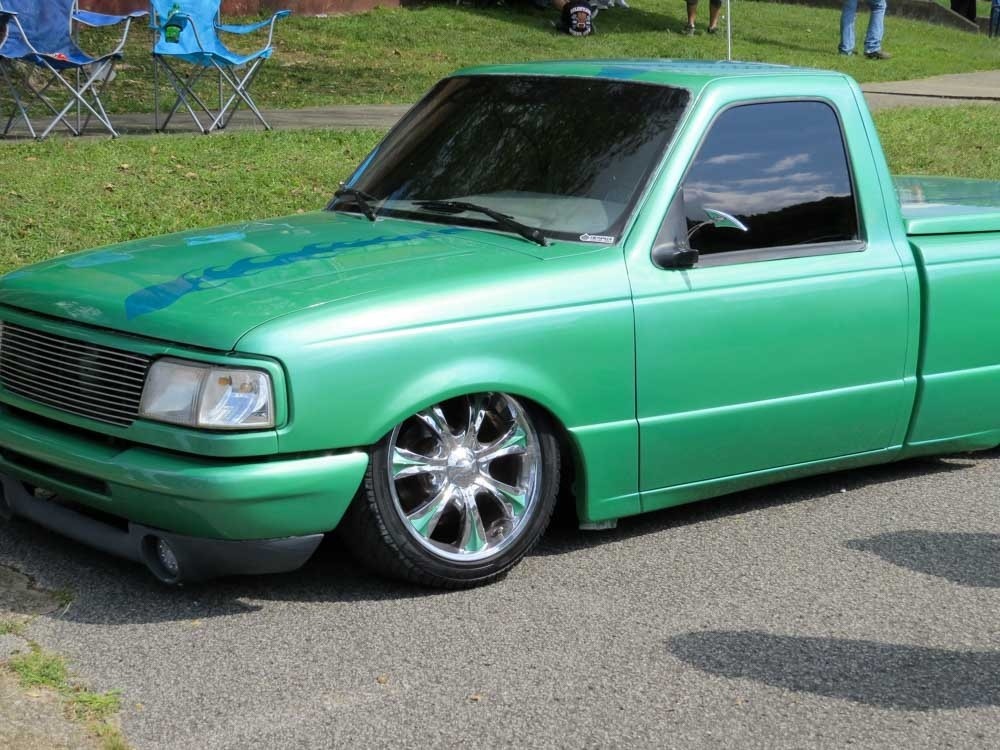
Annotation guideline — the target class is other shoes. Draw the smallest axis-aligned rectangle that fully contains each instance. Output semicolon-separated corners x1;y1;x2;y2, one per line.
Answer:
684;23;696;32
707;25;719;33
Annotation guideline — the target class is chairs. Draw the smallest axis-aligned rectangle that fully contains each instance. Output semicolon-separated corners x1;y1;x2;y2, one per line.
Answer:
0;0;150;142
148;0;292;136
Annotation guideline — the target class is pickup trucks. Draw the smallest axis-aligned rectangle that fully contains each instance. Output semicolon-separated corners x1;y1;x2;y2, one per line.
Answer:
0;1;1000;591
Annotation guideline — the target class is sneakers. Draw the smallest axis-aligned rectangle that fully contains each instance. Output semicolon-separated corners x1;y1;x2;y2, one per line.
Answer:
838;49;859;56
615;0;630;9
864;49;891;59
587;0;608;11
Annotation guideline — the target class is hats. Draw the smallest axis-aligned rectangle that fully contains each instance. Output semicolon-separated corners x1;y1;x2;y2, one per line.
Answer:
554;0;595;37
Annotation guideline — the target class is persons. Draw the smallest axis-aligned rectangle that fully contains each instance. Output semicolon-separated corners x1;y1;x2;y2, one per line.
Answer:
840;0;893;60
681;0;722;34
555;0;630;35
